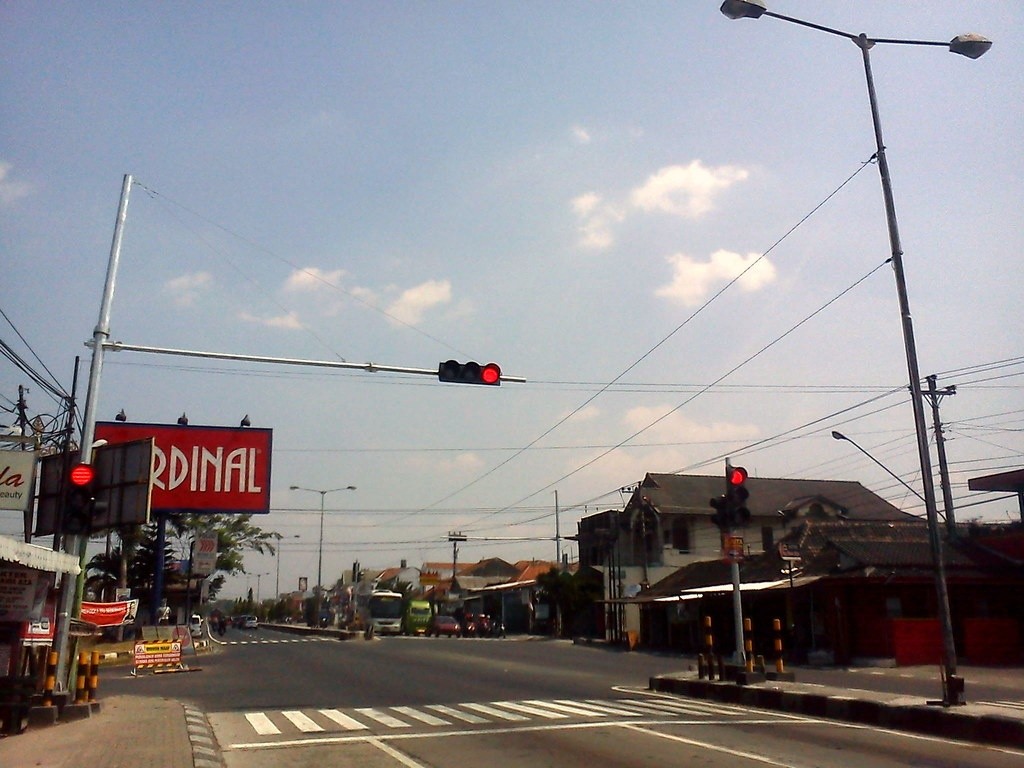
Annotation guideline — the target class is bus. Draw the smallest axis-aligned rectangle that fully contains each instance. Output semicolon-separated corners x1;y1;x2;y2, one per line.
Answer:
343;589;404;635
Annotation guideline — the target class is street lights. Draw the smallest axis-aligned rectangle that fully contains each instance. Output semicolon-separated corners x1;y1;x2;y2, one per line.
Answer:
290;484;357;588
718;1;995;702
243;533;302;605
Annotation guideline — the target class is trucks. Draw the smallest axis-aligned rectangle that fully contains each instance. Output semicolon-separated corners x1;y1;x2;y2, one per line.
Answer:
407;600;433;635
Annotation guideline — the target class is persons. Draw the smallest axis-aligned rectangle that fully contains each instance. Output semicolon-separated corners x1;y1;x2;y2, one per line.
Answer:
124;601;135;620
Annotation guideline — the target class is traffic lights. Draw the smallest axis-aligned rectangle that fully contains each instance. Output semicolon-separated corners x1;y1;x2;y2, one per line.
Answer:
710;496;729;533
65;463;96;533
438;360;503;385
728;465;752;529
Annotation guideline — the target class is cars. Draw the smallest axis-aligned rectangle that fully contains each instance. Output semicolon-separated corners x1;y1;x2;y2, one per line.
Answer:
206;608;331;636
428;611;505;638
